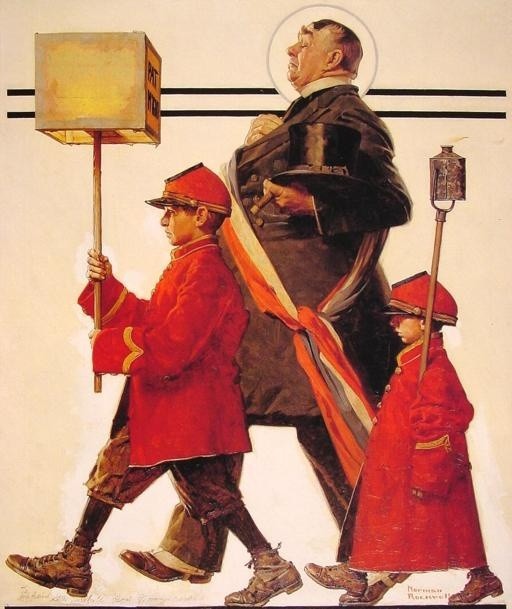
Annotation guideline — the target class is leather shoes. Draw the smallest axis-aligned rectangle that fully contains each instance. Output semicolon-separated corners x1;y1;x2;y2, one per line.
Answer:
119;550;211;584
451;566;504;605
303;562;411;606
224;551;302;606
5;540;92;596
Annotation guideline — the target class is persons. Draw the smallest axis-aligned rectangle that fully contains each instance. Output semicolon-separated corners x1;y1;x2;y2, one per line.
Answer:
4;161;303;608
117;15;414;593
303;270;504;605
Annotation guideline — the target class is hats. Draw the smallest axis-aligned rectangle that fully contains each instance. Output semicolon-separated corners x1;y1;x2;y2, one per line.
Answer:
378;272;457;325
272;122;371;195
145;163;232;217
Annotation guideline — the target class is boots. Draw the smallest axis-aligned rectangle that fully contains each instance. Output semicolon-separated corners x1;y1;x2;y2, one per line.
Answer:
284;591;286;592
